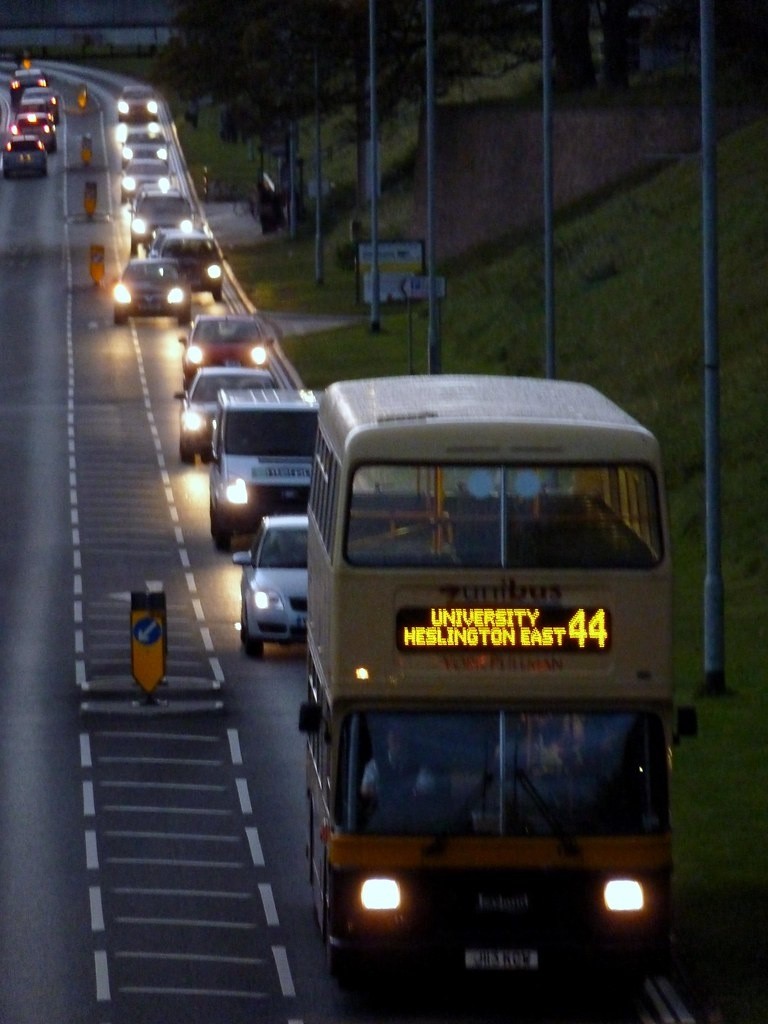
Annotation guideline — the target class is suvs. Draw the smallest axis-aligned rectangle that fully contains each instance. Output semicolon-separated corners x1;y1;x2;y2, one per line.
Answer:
201;385;319;549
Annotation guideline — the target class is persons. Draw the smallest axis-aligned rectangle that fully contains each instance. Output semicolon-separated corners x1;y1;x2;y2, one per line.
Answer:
361;719;444;805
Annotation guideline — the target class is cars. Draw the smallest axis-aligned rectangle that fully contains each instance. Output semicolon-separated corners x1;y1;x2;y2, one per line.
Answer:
20;87;59;121
150;226;224;300
174;313;272;394
128;187;194;260
14;109;57;152
118;123;171;169
116;83;158;123
230;513;313;659
120;157;170;205
173;365;285;466
3;133;49;177
111;257;196;324
10;67;47;105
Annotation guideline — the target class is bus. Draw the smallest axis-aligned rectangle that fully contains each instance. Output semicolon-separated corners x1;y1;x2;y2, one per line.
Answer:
297;369;705;1024
